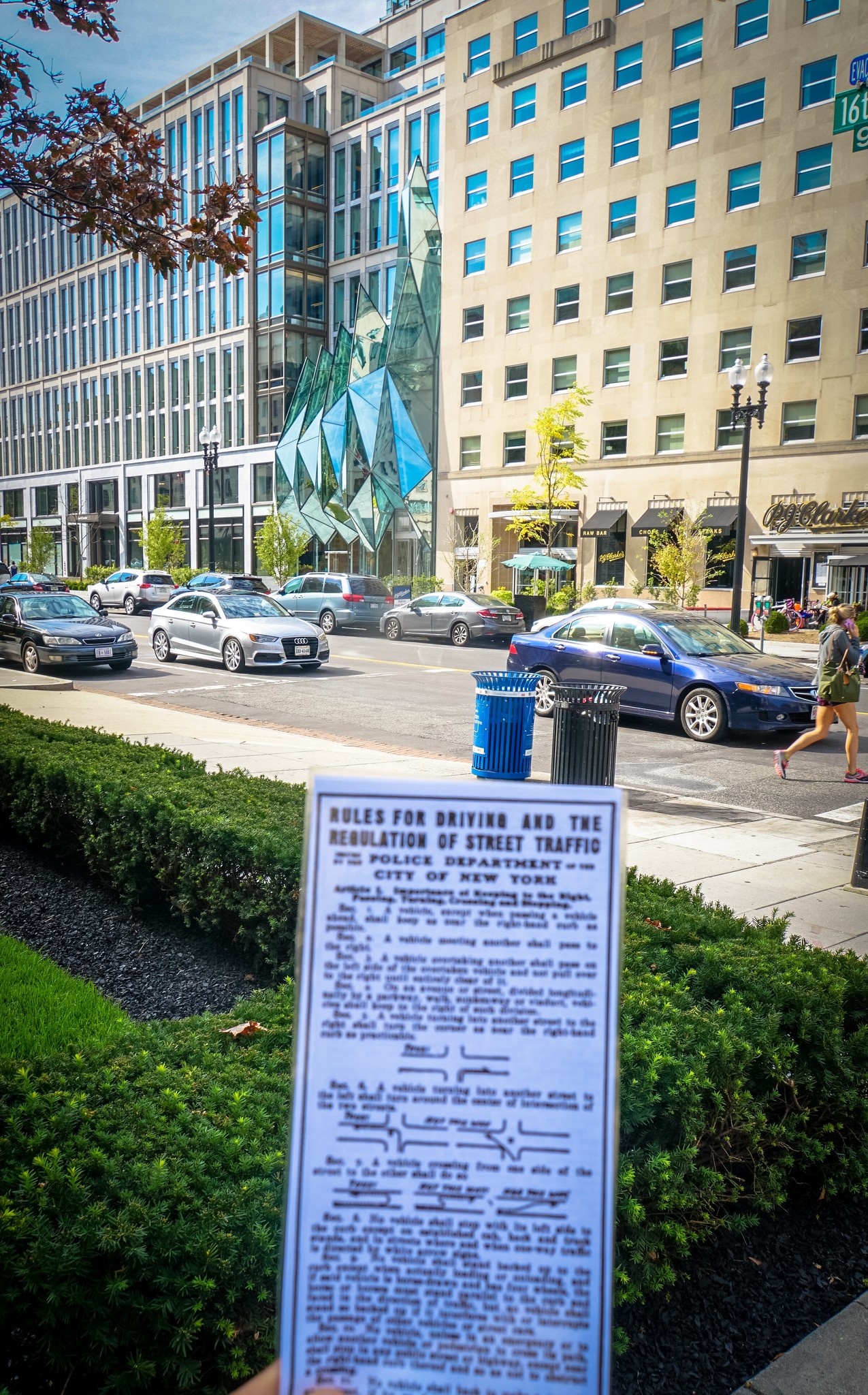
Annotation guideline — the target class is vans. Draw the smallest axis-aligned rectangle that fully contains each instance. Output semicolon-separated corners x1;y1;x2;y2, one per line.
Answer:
0;561;11;585
261;570;395;635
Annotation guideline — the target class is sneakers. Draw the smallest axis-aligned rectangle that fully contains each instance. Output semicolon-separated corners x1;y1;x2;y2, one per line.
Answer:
844;768;868;783
773;749;789;779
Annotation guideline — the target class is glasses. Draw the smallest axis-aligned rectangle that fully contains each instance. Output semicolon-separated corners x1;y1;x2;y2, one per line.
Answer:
846;617;858;622
807;583;809;584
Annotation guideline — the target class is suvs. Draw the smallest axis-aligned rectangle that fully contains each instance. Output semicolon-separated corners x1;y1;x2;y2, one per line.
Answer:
170;572;269;604
90;568;178;614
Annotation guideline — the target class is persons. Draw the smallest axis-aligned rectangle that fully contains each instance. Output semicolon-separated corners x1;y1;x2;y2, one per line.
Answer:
820;591;842;607
809;593;841;630
804;580;810;606
772;602;868;785
9;561;18;577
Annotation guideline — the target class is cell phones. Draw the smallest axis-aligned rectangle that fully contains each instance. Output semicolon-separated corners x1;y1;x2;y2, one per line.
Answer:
844;618;854;630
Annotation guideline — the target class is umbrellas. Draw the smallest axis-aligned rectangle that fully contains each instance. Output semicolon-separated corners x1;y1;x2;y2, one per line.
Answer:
498;551;576;596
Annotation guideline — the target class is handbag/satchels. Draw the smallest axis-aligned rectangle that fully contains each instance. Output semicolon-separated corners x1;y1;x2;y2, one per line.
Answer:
817;629;862;703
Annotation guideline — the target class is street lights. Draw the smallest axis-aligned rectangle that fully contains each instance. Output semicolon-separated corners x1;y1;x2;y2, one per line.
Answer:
729;352;773;633
199;425;222;572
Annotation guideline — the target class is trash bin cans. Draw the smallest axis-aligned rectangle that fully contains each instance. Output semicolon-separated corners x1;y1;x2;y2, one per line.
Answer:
470;669;543;780
548;681;628;787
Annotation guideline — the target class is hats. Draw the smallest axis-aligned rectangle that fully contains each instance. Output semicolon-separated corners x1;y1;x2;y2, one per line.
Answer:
827;592;836;599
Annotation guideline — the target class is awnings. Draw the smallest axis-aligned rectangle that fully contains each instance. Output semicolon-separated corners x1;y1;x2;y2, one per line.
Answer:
631;507;683;537
579;510;627;538
691;505;740;538
821;553;868;567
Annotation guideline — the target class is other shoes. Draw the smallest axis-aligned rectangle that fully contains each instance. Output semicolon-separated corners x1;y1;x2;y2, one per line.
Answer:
808;621;819;625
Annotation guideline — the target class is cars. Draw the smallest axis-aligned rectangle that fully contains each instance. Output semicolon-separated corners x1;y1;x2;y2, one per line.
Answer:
380;591;525;647
0;572;70;593
148;588;330;673
0;591;138;673
532;598;720;650
506;609;839;743
